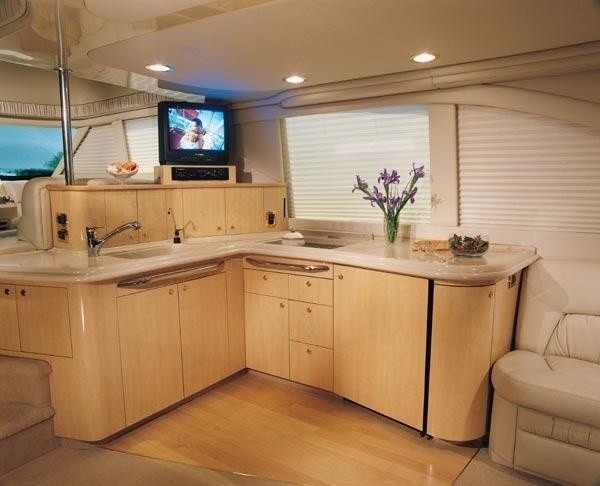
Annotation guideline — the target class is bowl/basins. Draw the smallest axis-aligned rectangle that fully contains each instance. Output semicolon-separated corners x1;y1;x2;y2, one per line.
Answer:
447;241;490;258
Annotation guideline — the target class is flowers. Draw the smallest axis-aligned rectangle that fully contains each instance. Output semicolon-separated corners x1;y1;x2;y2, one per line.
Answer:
348;160;428;245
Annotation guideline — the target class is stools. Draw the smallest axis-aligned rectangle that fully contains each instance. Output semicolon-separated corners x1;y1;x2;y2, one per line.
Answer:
0;354;63;480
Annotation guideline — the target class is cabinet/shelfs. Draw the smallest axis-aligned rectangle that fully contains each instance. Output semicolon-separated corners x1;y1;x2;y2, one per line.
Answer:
334;256;432;440
46;178;290;250
240;247;333;397
95;252;250;445
0;272;74;361
422;267;527;445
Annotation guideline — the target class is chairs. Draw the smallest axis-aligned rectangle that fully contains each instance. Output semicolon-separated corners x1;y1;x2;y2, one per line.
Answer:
485;248;600;486
0;175;62;247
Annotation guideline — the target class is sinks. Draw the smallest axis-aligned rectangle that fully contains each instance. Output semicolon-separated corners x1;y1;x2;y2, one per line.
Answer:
103;245;192;260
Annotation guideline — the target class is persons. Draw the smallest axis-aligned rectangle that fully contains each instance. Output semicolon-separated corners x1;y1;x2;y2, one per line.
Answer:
176;118;214;150
175;130;201;151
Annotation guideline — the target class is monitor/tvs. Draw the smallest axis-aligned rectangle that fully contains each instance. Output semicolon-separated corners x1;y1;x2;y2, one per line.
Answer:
158;101;229;165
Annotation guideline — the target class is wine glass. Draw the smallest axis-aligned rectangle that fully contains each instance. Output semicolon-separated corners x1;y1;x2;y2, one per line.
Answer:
107;165;139;186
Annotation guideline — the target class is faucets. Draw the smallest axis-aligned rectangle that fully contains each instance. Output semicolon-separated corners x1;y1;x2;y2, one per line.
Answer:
166;207;184;244
84;220;142;256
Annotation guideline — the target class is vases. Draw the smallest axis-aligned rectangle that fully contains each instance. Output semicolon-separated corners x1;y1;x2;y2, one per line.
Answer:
381;209;401;247
